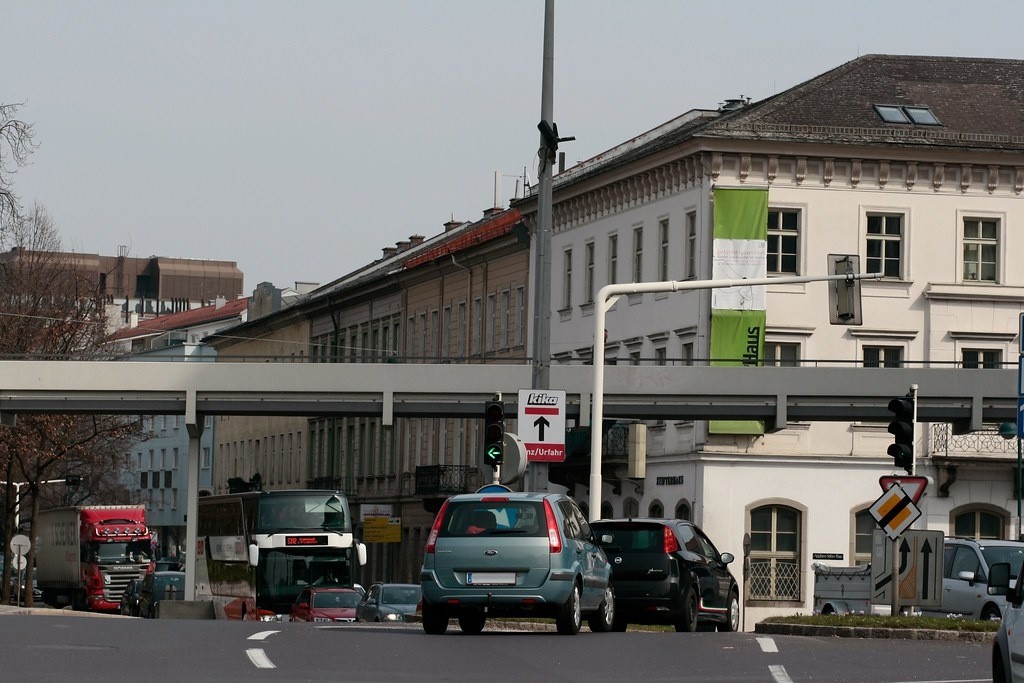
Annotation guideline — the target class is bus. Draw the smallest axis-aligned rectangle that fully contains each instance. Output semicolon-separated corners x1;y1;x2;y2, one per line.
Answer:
193;487;368;620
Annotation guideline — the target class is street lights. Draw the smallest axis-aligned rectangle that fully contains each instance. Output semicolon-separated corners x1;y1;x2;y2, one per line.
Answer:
1;477;84;535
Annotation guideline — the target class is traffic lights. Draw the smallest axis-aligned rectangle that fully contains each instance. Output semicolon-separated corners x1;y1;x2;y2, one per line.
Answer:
482;399;506;467
887;395;916;469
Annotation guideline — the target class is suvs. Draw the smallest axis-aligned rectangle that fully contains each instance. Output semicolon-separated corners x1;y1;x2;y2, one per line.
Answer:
587;516;741;631
941;534;1024;624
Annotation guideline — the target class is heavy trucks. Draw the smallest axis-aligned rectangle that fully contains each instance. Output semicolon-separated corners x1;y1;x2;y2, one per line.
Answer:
35;504;154;612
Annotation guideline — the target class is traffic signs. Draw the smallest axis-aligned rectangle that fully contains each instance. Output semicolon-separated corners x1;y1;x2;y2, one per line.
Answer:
517;387;567;463
364;516;402;544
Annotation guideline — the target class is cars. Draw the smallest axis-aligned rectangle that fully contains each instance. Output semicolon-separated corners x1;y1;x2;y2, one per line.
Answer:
122;559;185;617
990;560;1024;683
291;581;363;623
355;581;422;621
15;567;43;601
420;491;617;635
